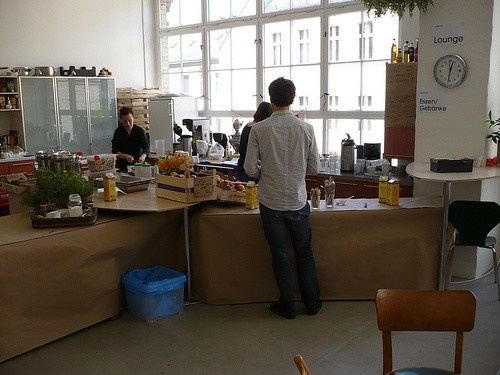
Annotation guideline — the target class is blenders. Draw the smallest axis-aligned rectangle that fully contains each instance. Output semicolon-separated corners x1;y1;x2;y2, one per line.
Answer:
173;118;211;159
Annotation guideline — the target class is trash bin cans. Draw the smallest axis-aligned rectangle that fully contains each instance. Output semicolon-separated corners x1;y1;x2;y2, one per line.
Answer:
122;266;186;322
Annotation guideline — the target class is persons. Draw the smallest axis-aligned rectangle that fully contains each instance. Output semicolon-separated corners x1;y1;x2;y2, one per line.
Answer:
111;107;149;171
244;77;323;320
234;102;273;183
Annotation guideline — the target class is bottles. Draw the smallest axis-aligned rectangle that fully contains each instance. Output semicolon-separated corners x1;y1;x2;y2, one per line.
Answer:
390;37;419;64
134;163;159;178
40;202;48;217
5;96;13;109
35;150;80;174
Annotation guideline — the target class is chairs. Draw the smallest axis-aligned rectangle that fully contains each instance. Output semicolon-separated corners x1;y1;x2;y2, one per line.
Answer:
375;289;476;375
446;201;500;303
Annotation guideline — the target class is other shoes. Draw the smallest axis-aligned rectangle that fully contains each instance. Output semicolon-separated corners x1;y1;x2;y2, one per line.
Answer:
270;302;296;319
307;301;322;315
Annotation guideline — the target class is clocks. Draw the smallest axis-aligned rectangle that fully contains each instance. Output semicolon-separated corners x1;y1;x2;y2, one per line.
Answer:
434;54;468;88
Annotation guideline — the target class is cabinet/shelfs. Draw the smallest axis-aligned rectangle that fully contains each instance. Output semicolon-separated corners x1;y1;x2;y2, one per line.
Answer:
0;76;118;176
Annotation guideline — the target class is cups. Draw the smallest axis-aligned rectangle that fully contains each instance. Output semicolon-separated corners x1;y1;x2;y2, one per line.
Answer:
310;187;321;209
67;194;83;218
317;151;341;173
323;179;336;208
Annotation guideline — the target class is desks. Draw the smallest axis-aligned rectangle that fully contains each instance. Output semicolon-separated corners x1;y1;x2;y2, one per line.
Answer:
406;161;500;291
0;192;185;367
192;195;445;307
83;176;216;308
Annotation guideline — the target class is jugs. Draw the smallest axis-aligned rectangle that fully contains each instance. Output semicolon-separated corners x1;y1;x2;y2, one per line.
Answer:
173;124;194;157
153;139;167;156
341;133;354;174
195;139;208;160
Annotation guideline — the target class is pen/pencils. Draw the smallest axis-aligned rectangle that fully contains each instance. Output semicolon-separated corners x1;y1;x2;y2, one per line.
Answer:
365;202;367;208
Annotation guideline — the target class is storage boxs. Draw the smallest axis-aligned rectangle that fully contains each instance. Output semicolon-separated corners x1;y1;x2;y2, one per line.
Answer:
155;168;217;203
431;157;473;173
60;66;96;77
